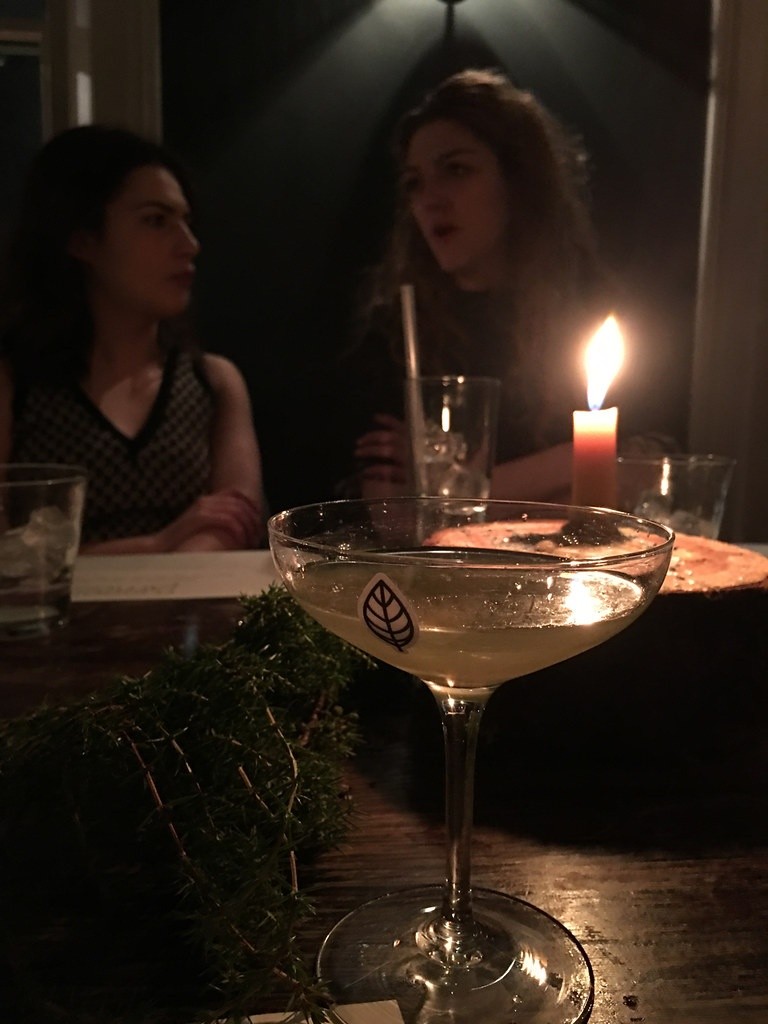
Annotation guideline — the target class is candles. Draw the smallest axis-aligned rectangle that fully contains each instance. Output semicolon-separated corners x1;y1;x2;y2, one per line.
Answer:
568;310;628;521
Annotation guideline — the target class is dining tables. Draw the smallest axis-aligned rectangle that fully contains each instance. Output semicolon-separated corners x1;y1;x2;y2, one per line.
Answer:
0;550;768;1024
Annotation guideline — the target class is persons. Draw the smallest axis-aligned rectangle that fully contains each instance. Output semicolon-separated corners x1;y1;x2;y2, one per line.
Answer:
0;125;265;551
357;69;681;544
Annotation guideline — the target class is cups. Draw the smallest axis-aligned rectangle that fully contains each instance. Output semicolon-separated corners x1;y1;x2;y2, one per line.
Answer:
0;463;94;633
400;371;504;545
614;451;735;561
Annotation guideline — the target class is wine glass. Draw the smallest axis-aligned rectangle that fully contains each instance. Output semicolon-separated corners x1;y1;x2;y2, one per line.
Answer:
268;494;676;1024
